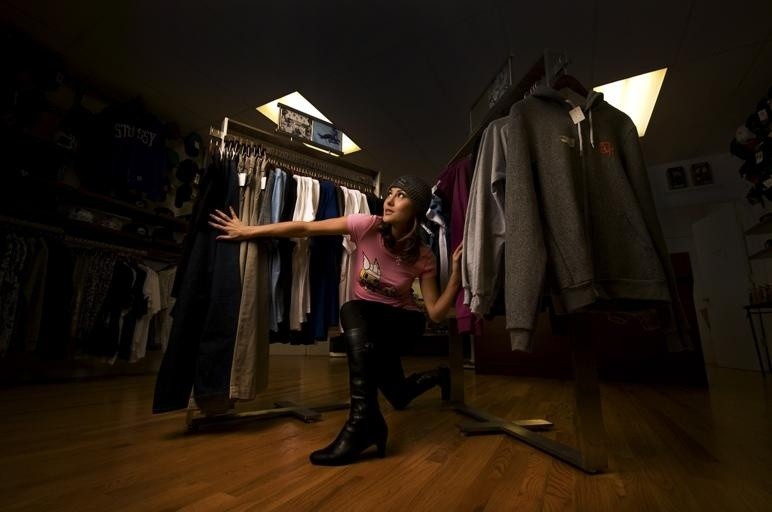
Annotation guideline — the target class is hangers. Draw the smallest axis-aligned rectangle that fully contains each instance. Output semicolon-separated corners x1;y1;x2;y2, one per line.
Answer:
205;127;379;198
522;53;589;111
0;217;181;269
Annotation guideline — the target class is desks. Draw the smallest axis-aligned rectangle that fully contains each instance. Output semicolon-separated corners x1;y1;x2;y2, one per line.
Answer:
744;302;772;376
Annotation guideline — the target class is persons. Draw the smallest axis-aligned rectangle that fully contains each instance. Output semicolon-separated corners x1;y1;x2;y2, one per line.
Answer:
208;174;463;467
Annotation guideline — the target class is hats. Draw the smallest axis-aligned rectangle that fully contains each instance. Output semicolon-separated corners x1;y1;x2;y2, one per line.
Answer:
387;175;432;215
730;85;772;208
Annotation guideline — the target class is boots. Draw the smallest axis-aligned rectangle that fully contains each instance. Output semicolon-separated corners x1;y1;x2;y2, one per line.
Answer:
308;327;388;465
404;364;451;402
